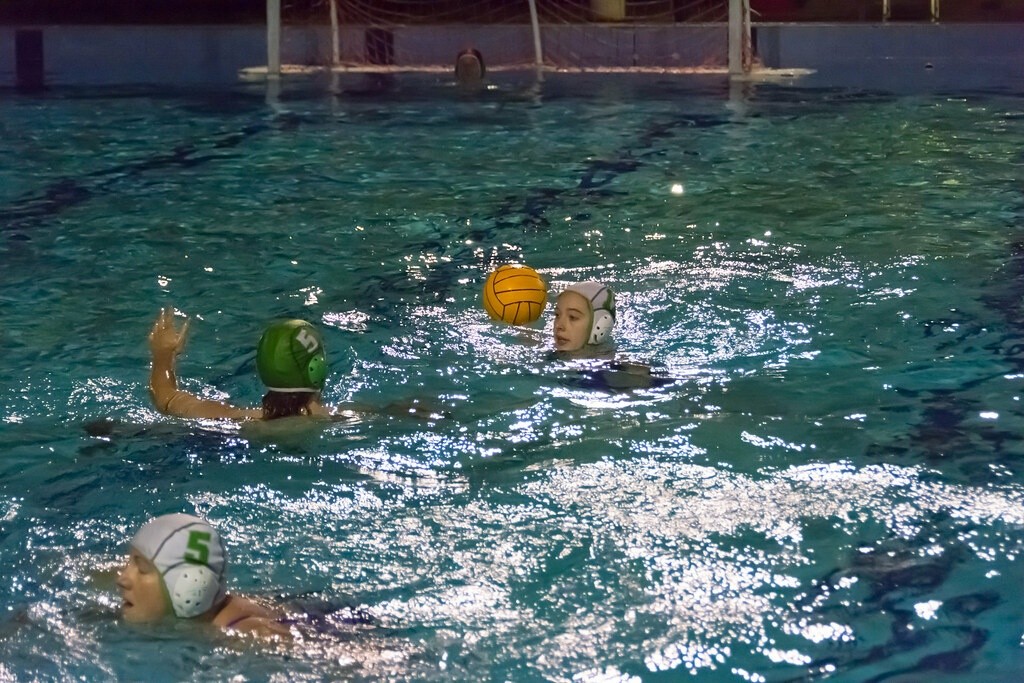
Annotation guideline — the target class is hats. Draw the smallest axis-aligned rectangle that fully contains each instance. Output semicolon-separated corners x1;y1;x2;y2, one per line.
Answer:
455;48;484;79
131;513;226;618
547;279;616;345
256;319;326;393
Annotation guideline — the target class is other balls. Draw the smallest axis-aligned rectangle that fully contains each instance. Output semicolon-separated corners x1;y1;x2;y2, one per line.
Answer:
482;261;548;327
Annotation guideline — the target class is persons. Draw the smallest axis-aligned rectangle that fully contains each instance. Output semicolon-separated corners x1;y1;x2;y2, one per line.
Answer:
539;280;657;391
9;511;467;660
438;47;496;101
146;304;445;457
